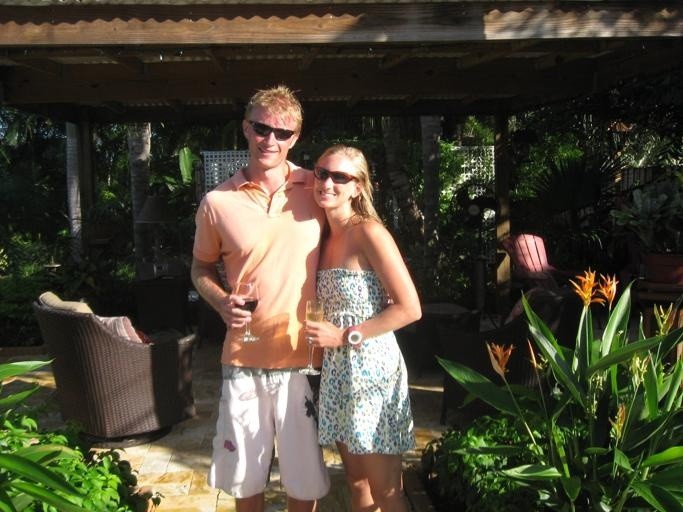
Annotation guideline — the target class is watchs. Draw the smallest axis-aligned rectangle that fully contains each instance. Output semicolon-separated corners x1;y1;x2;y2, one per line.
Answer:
348;325;363;350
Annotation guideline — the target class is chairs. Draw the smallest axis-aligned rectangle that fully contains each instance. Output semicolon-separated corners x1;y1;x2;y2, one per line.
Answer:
417;233;634;425
31;301;196;448
636;253;683;337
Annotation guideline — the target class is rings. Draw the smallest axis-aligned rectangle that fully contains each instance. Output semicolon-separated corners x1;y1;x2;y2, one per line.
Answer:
308;337;312;345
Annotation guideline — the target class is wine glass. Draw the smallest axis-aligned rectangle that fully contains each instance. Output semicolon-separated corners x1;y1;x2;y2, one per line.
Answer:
234;280;260;343
299;299;324;376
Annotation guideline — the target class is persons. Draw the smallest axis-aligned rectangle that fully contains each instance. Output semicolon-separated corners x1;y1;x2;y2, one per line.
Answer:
190;85;330;512
304;143;422;512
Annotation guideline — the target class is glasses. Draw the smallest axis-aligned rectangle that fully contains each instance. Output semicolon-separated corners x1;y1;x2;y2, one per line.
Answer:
313;166;359;185
245;118;295;141
